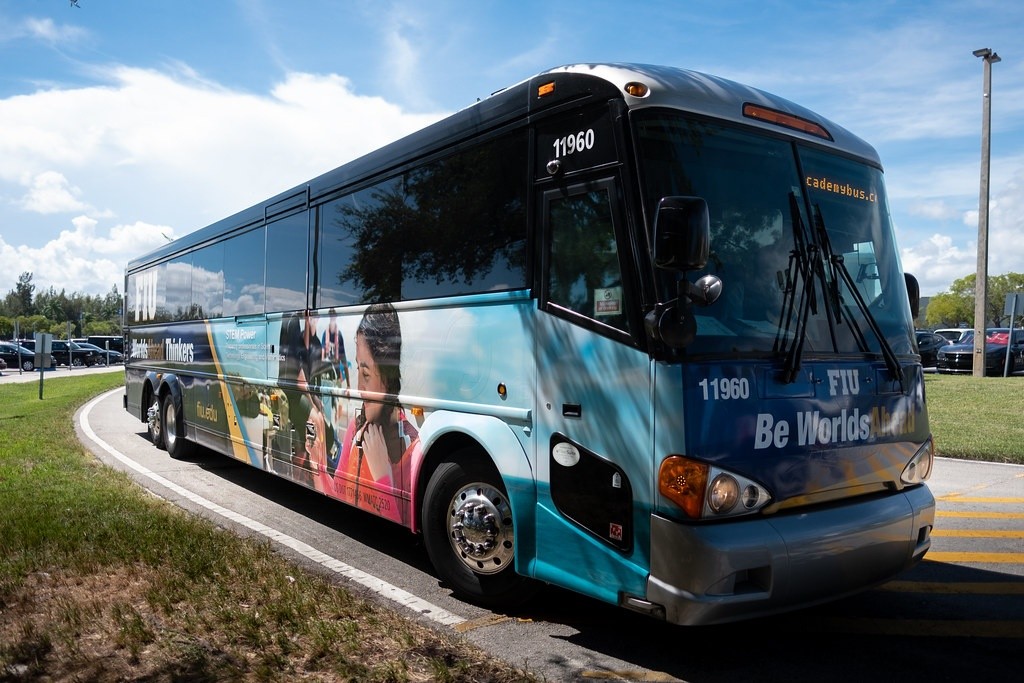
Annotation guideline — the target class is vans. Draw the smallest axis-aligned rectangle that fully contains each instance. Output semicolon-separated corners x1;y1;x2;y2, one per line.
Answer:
21;339;102;367
88;335;124;355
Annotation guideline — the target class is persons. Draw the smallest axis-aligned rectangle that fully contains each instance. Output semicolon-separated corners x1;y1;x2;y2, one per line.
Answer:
278;304;423;534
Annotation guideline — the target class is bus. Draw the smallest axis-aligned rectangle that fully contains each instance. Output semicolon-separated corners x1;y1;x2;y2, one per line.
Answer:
122;61;936;630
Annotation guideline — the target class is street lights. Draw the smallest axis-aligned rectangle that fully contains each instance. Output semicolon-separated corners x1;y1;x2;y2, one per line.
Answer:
972;46;1002;380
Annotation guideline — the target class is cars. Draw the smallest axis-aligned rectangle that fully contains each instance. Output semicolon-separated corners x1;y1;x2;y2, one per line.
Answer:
0;358;7;369
916;332;945;368
936;327;1024;376
0;340;58;372
77;343;122;365
934;327;989;347
